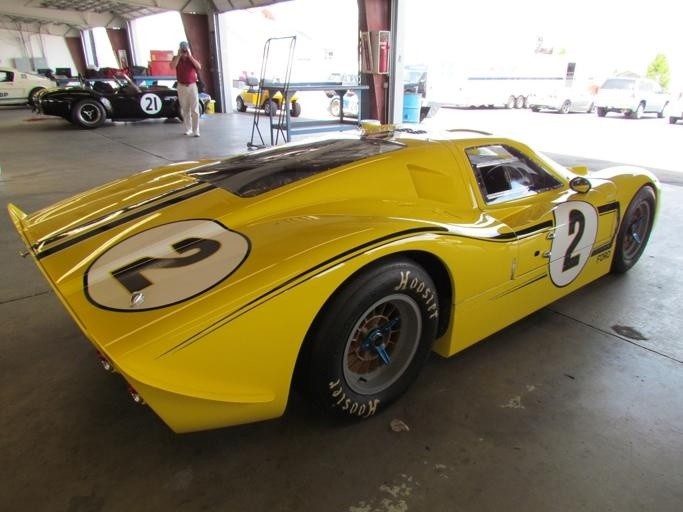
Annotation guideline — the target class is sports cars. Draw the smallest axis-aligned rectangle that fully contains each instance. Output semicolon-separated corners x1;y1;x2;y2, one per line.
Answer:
6;119;663;435
31;70;213;130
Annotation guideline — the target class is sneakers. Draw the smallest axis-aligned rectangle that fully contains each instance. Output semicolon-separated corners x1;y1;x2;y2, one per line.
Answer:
184;130;200;136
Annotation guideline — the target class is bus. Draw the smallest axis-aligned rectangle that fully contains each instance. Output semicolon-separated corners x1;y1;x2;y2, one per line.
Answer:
440;59;599;110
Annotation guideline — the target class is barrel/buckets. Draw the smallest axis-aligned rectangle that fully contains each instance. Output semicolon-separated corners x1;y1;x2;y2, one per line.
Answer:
403;92;421;122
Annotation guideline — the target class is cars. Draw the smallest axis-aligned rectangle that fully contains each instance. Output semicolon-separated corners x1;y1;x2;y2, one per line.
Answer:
0;66;58;108
327;89;432;122
527;74;683;124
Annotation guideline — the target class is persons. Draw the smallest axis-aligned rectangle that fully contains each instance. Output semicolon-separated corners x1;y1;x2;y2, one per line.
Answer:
169;40;202;139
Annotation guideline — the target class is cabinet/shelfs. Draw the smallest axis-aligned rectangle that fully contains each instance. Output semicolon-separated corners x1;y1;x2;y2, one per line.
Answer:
258;81;370;146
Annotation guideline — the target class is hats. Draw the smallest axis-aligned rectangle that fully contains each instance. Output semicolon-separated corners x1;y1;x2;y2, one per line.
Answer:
180;42;188;47
